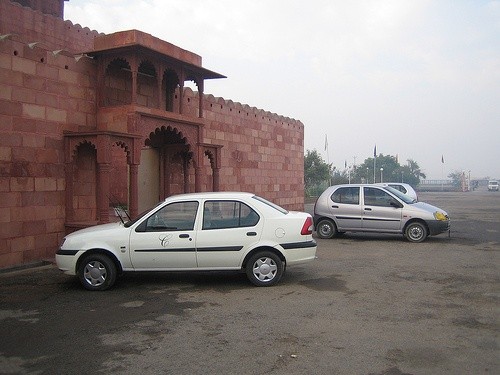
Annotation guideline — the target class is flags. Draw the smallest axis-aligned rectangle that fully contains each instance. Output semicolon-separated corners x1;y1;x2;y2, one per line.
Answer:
374;144;377;158
441;155;445;164
324;135;329;150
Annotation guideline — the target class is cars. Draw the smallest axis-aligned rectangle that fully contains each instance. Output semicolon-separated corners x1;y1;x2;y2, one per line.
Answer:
377;181;419;204
314;183;452;242
53;191;317;289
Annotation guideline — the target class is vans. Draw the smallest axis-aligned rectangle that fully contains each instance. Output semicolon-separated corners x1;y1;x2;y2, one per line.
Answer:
489;180;499;191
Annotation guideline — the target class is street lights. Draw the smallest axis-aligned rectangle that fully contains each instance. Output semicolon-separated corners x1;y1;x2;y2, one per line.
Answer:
401;171;404;182
379;168;384;183
366;167;369;185
349;164;351;184
